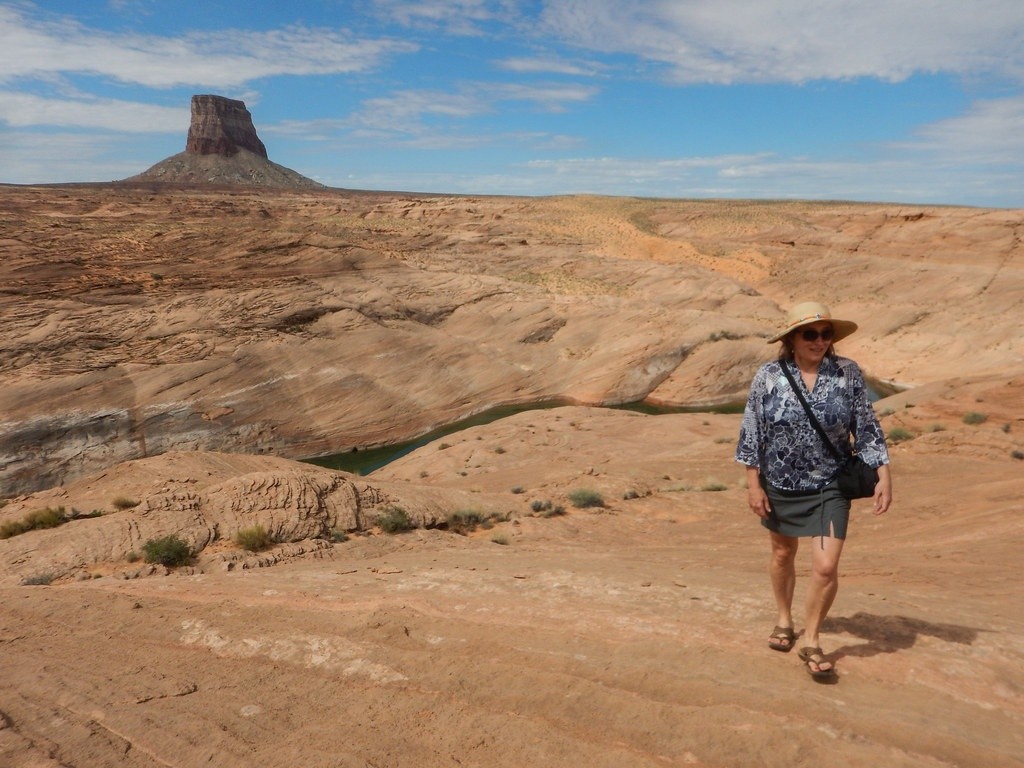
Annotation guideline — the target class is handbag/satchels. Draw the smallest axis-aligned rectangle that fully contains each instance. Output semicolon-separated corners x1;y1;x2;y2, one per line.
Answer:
838;456;880;498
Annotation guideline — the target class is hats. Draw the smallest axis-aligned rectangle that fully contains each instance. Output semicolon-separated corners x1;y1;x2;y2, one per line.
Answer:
767;301;858;344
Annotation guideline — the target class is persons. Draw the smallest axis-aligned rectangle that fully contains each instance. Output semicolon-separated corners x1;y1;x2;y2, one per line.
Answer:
735;302;892;683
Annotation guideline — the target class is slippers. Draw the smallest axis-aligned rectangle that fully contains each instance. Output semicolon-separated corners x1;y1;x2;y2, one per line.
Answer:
797;647;833;677
768;625;794;650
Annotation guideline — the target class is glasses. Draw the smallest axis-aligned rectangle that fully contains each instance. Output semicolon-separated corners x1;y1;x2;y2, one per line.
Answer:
793;325;836;342
